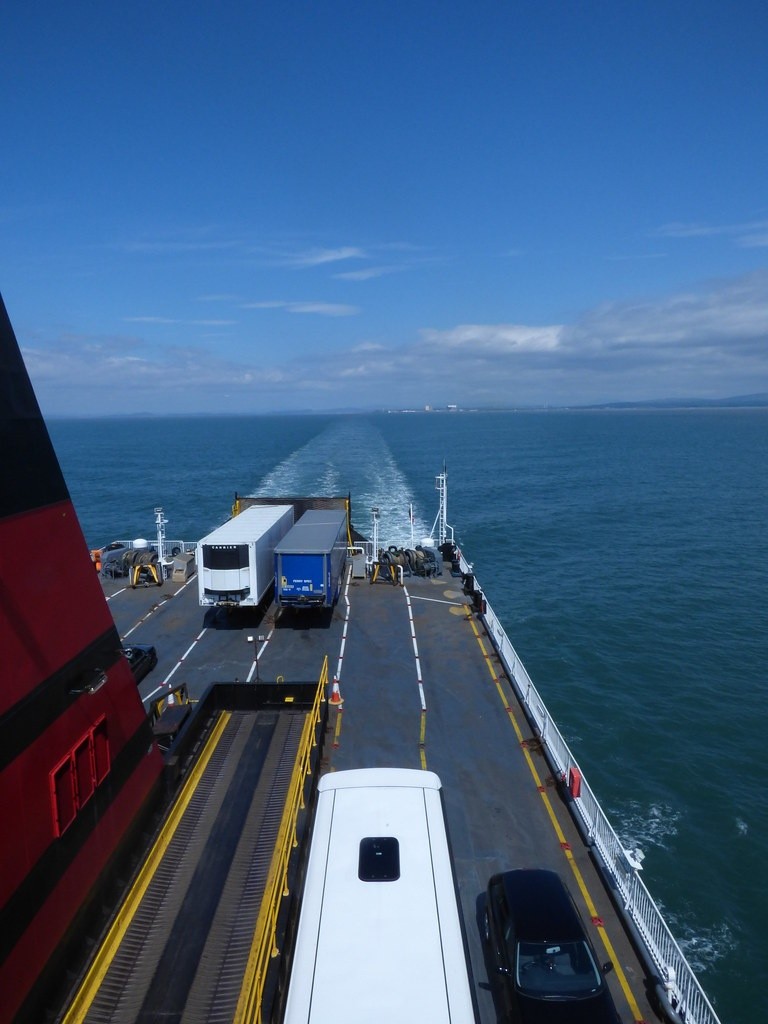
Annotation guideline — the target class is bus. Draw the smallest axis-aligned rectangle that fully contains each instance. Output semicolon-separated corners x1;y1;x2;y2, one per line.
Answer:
280;767;477;1024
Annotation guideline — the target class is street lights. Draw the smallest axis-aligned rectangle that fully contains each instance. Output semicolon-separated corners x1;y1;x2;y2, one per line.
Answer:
247;635;265;683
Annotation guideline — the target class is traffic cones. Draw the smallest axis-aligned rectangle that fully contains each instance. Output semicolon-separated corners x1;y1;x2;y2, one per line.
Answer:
328;674;345;705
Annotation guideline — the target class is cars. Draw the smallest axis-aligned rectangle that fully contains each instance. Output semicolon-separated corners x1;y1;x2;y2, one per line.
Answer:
122;643;157;684
482;867;614;1014
